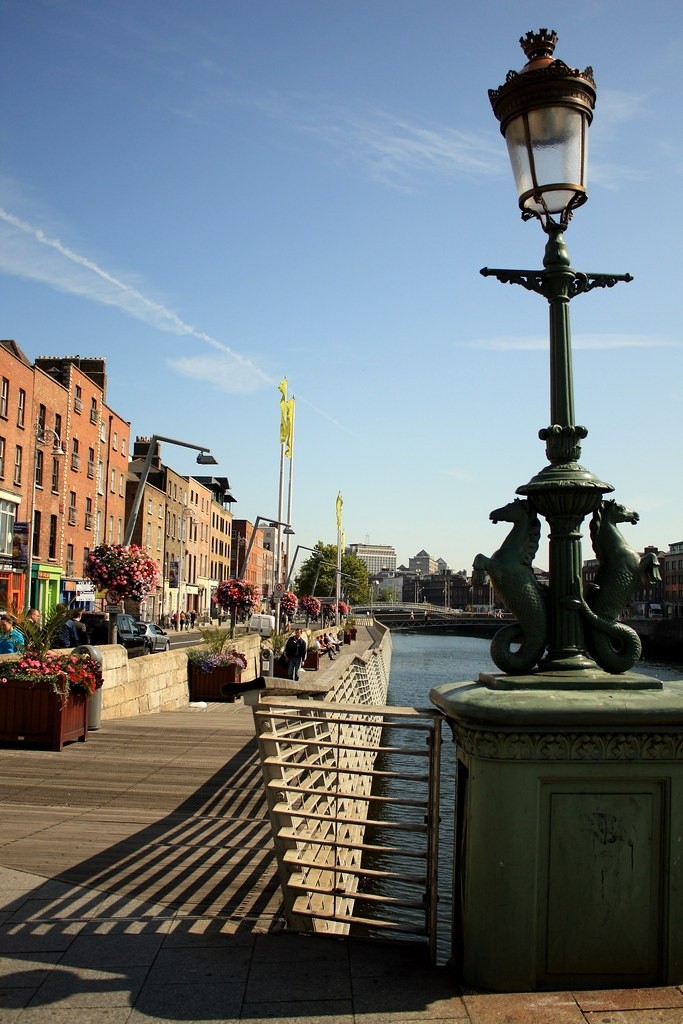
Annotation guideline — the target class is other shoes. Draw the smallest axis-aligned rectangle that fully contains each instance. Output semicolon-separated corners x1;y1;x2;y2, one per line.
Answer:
330;657;335;660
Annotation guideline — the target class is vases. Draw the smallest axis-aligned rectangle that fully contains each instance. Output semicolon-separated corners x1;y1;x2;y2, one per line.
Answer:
0;674;88;752
189;664;241;703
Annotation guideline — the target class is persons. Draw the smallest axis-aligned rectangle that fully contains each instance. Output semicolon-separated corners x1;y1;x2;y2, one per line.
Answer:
284;628;306;681
0;604;90;654
316;633;340;661
170;610;253;630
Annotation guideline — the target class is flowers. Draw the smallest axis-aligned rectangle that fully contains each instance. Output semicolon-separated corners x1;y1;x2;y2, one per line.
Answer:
84;541;161;604
185;646;248;674
330;600;349;615
269;590;299;619
299;595;321;622
212;577;260;618
0;642;104;712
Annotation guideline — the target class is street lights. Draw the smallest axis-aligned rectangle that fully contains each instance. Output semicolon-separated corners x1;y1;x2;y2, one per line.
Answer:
416;587;424;603
478;27;664;677
108;434;218;644
23;429;67;614
469;587;473;612
369;580;380;606
230;515;295;639
286;545;359;628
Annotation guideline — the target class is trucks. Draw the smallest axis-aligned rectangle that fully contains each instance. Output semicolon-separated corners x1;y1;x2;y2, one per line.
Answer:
632;602;663;618
247;614;275;637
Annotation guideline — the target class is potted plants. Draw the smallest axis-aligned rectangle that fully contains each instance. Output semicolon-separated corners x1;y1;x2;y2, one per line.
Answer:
343;615;357;646
301;630;320;671
260;629;294;678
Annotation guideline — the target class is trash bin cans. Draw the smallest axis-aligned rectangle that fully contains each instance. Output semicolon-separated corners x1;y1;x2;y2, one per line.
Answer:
338;630;344;646
260;649;274;677
71;645;101;730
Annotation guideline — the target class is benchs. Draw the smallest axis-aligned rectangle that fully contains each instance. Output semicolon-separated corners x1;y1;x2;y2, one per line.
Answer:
318;632;342;657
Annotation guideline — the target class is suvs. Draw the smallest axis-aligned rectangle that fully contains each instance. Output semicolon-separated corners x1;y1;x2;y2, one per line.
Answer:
81;611;154;659
137;620;171;654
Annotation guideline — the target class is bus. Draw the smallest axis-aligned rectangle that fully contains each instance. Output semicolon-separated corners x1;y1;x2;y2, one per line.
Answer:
472;605;492;612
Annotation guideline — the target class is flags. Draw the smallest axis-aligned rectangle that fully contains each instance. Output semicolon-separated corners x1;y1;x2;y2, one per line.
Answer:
335;496;346;554
277;378;293;457
